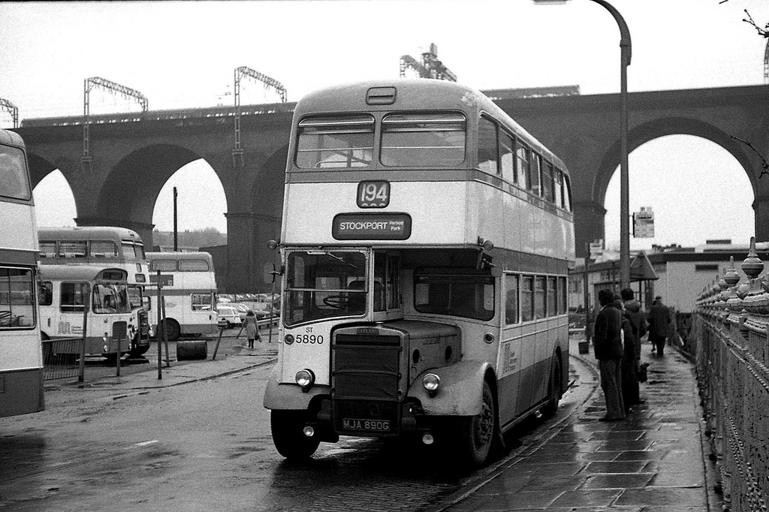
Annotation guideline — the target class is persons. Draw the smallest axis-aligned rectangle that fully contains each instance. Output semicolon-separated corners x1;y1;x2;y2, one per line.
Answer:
241;310;259;350
650;296;671;357
103;285;113;308
647;301;657;352
593;288;647;422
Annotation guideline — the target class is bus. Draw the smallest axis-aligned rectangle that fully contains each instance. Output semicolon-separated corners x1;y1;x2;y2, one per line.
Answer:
261;78;577;468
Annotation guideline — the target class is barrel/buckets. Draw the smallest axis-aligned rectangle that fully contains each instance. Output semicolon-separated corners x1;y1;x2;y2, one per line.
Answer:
579;341;589;353
176;339;207;361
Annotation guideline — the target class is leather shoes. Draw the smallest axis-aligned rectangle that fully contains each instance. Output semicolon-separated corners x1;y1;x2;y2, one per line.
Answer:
599;418;615;422
605;413;625;421
